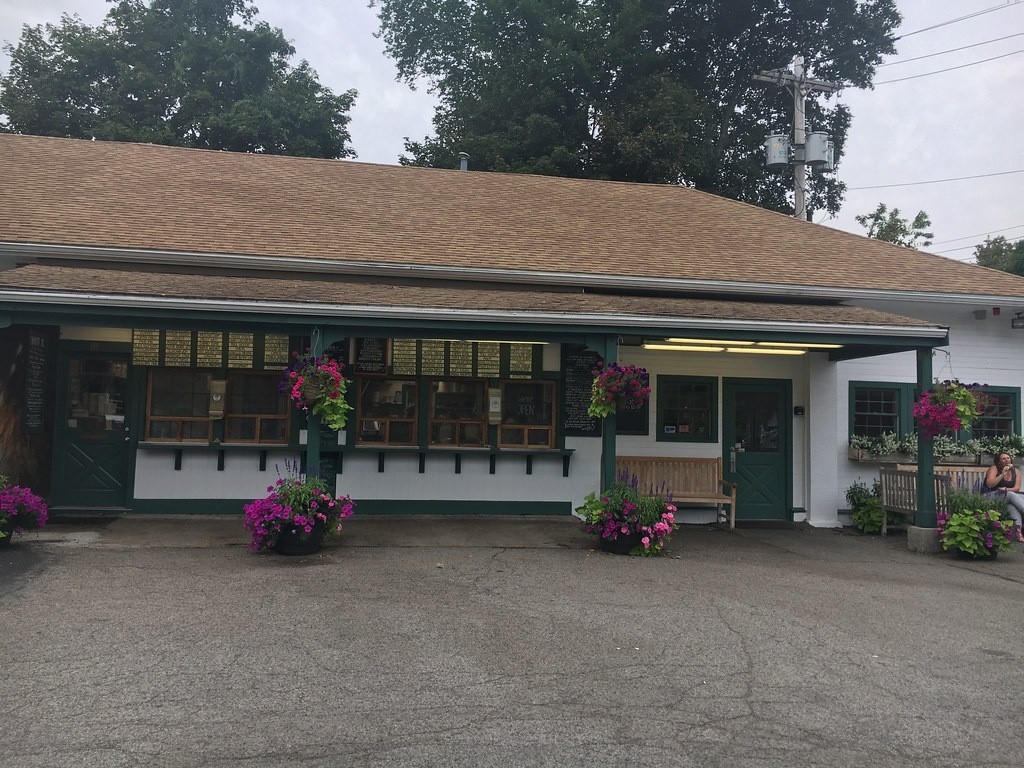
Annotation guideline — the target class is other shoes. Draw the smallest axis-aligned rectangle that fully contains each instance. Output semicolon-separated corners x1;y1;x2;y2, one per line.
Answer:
1015;530;1024;542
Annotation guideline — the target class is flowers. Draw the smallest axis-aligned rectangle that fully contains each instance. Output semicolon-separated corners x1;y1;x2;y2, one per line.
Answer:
0;475;49;539
573;465;677;557
277;346;356;431
243;455;356;553
935;488;1020;558
587;361;651;419
912;378;997;442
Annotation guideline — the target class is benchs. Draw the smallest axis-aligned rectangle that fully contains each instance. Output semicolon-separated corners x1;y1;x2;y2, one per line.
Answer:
897;464;990;518
879;467;951;540
614;456;738;529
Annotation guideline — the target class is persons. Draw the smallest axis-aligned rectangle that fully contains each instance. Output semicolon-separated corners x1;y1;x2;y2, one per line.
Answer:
981;453;1024;542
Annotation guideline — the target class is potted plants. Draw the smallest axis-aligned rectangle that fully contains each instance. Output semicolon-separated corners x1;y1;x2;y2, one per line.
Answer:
850;432;1024;462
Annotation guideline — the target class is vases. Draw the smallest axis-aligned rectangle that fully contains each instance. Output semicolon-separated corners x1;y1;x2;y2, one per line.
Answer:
0;522;13;544
278;520;323;555
599;530;642;555
956;544;998;561
